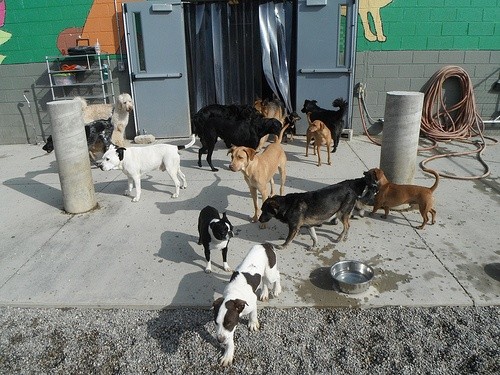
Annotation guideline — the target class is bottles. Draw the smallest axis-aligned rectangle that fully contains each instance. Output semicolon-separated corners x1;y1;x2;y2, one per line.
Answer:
94;38;100;55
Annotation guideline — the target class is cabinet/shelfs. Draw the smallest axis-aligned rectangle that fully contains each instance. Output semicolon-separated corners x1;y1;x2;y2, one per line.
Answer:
45;52;116;105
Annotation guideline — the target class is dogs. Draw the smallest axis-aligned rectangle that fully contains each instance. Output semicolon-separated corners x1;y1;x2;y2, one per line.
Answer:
96;144;187;202
284;113;301;142
73;92;133;148
363;167;440;230
192;104;283;172
225;118;297;222
212;243;282;368
197;206;234;273
260;178;369;249
42;117;114;165
252;98;285;151
305;112;333;166
301;97;349;153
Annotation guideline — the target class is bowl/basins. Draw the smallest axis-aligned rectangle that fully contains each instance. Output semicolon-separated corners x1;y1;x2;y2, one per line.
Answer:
329;260;374;294
53;73;76;85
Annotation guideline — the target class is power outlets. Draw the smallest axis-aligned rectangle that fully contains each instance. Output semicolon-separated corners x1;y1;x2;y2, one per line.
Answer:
358;90;365;98
118;62;126;72
